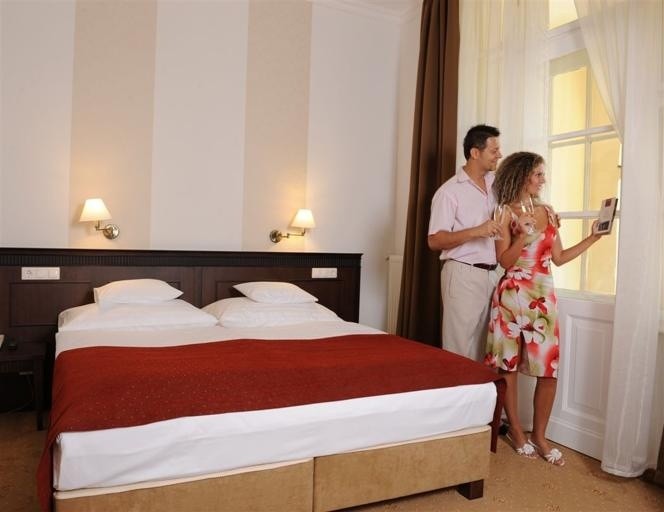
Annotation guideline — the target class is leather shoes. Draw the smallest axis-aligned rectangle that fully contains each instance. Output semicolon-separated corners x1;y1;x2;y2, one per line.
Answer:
498;421;510;435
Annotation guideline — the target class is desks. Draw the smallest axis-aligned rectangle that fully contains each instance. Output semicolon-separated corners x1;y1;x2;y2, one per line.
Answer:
0;339;46;432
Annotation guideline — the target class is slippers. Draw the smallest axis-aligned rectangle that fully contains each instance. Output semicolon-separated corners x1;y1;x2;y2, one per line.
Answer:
528;440;565;467
506;433;539;460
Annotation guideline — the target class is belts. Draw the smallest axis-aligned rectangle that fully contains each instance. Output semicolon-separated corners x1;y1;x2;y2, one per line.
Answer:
474;263;499;271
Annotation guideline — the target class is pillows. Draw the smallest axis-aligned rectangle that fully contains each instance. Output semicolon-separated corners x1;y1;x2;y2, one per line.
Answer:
56;277;343;334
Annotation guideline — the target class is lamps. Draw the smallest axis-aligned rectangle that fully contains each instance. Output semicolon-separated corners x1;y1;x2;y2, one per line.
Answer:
78;198;119;239
269;209;315;243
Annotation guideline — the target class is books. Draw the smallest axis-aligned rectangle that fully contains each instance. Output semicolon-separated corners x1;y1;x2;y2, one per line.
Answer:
595;197;618;235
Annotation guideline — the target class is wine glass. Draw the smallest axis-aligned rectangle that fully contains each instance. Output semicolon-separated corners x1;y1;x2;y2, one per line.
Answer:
490;206;506;242
520;197;536;234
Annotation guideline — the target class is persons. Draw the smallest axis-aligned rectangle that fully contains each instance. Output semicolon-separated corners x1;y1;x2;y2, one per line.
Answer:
483;149;608;467
426;124;562;368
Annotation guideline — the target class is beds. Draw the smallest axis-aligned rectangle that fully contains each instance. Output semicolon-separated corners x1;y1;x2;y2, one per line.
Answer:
49;250;499;512
